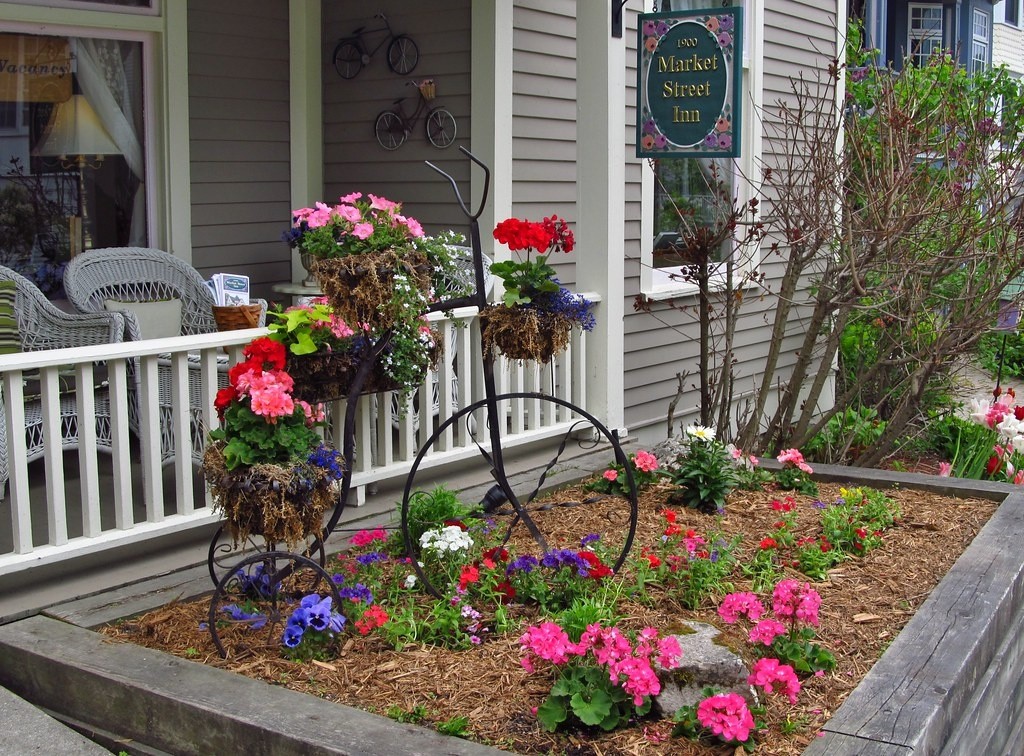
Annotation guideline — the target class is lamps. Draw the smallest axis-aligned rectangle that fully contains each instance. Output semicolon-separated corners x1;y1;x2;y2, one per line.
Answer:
30;94;124;252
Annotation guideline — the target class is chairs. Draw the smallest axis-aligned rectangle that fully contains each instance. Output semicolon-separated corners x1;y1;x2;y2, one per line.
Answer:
351;242;495;465
0;265;126;499
63;246;268;506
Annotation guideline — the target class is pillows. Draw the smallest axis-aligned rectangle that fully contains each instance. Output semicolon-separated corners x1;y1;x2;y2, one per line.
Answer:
103;299;183;341
0;279;23;356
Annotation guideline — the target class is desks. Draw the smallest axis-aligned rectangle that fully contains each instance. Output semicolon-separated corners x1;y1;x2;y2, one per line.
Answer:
145;346;232;471
272;284;325;297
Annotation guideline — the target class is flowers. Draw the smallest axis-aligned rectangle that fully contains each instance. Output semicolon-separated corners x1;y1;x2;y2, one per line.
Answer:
201;424;904;746
266;297;444;403
196;337;350;579
937;386;1024;485
266;192;477;422
280;217;316;255
475;213;598;369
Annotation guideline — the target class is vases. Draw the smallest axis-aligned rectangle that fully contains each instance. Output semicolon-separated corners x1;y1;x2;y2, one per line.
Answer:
315;265;429;315
226;492;310;537
496;327;552;360
301;252;321;286
